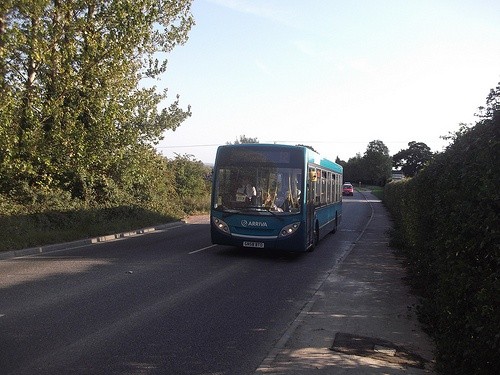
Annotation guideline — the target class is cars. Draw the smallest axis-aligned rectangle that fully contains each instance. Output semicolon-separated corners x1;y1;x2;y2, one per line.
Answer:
342;183;354;196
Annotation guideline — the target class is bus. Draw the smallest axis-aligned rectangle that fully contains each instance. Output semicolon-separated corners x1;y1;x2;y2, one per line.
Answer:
210;145;344;254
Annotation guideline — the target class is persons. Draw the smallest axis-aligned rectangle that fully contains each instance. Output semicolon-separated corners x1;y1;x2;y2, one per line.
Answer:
222;173;342;219
